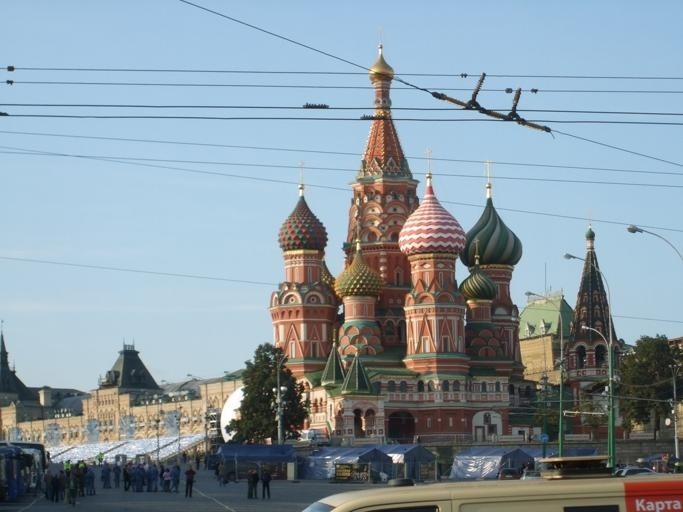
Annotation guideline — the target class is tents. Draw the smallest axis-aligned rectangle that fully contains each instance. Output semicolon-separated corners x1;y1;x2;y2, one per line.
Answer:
302;445;392;483
362;443;438;484
214;442;294;480
447;446;534;482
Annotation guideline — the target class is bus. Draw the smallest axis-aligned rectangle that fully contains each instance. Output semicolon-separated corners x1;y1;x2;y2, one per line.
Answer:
300;455;683;512
0;442;46;501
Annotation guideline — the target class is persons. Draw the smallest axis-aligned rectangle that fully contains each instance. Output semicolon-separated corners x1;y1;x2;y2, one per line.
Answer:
260;468;271;500
194;451;200;468
184;463;196;498
247;469;254;499
95;450;105;467
42;458;180;505
182;450;186;462
217;461;226;487
251;469;259;499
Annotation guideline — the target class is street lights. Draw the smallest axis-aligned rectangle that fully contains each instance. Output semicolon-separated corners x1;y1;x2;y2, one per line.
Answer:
272;353;289;444
524;225;683;476
161;374;208;456
